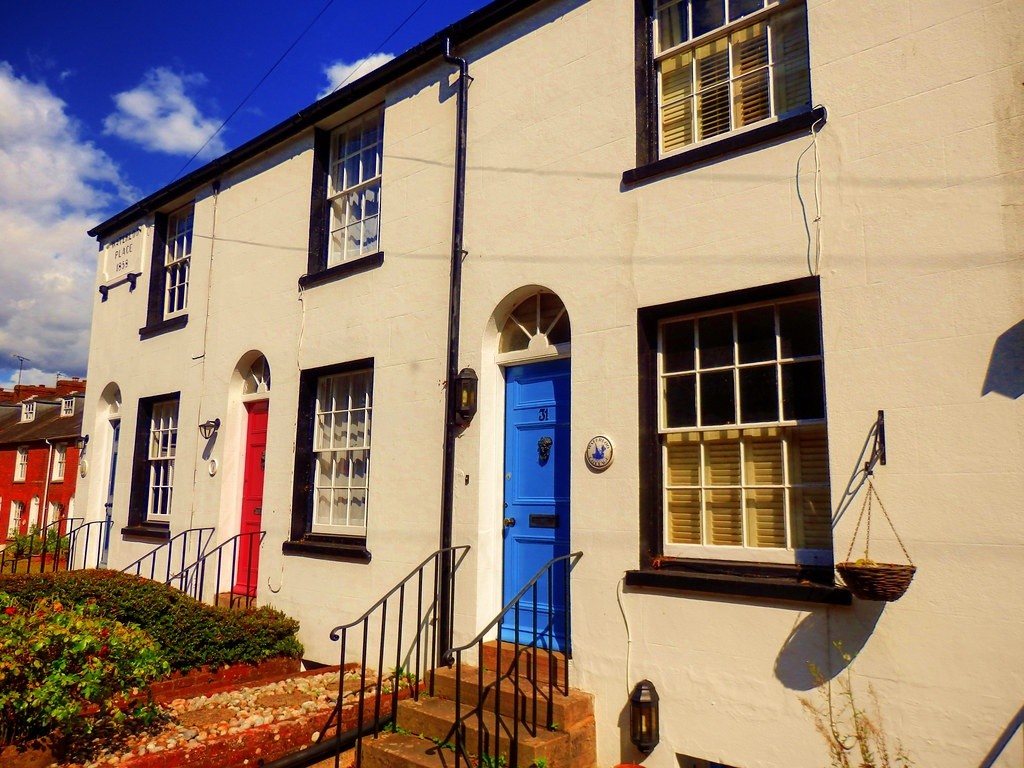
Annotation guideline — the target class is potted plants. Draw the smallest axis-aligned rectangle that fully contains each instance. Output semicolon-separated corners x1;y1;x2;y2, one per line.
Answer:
9;522;68;565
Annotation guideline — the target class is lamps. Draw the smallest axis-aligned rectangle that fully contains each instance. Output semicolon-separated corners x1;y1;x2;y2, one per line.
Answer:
200;418;220;440
628;681;662;758
457;367;477;422
74;435;89;449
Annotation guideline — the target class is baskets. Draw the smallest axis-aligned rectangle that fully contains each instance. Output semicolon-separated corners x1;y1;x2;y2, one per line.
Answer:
837;562;916;602
